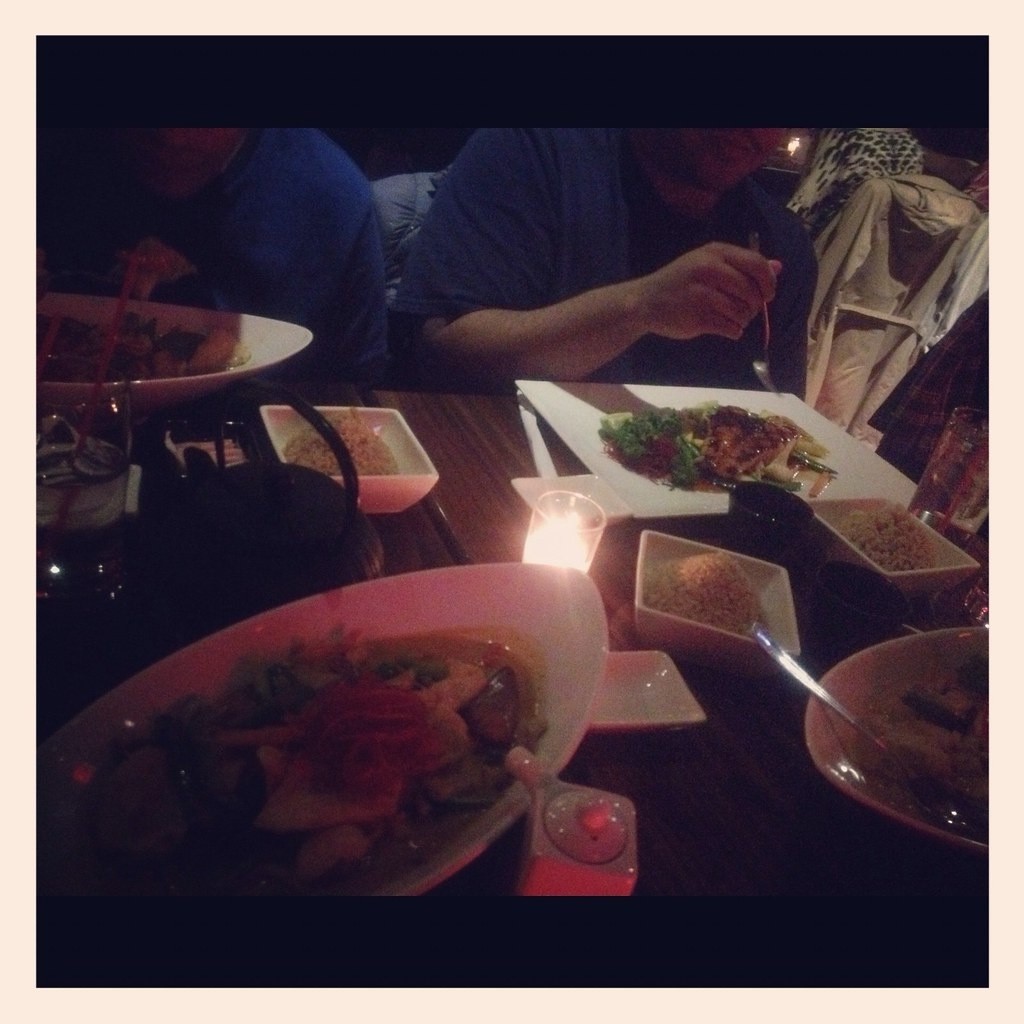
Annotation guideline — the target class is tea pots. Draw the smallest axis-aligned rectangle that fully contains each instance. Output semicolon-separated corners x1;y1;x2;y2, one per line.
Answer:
177;378;384;642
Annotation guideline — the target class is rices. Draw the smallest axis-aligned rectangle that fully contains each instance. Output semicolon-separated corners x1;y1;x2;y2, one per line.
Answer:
643;554;768;638
286;409;398;477
834;503;933;571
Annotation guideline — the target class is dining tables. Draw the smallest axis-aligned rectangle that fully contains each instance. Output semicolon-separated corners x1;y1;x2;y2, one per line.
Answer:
267;384;988;894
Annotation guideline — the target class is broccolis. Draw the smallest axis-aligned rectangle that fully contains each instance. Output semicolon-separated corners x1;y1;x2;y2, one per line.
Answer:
615;410;695;486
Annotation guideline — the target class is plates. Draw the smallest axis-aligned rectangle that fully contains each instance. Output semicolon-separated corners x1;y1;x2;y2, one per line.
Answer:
587;648;707;732
37;290;314;406
37;561;606;897
515;377;920;511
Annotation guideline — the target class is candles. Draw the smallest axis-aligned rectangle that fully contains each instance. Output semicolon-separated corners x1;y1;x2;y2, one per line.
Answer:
522;490;607;574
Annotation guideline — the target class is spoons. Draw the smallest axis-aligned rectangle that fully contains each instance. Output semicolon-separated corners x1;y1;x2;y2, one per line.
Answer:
748;232;788;395
751;624;987;825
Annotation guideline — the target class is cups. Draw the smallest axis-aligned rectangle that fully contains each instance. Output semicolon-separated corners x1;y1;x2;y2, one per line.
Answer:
907;407;989;549
37;359;131;607
498;745;640;897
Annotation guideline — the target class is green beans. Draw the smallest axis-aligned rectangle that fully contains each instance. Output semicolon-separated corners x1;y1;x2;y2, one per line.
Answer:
795;455;838;475
757;479;802;490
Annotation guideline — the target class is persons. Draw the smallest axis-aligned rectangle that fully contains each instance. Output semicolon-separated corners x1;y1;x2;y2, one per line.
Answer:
38;130;389;396
389;123;819;395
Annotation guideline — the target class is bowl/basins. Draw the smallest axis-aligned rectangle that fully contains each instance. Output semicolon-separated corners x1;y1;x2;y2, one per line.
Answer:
634;527;802;681
807;498;981;598
805;627;989;851
258;403;440;515
